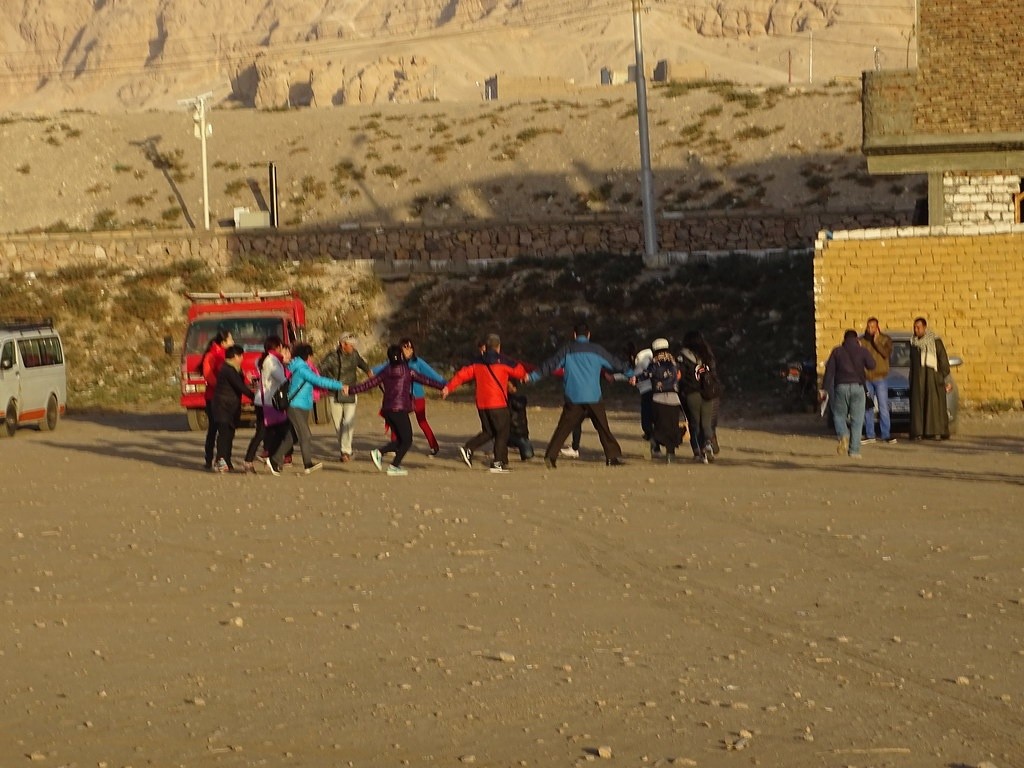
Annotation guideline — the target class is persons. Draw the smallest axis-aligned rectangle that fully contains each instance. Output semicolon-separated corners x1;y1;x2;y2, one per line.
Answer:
909;316;952;441
451;334;520;471
671;329;720;464
212;345;255;473
265;343;347;476
370;337;447;458
441;333;526;473
523;361;613;459
284;347;329;424
316;331;373;462
610;347;679;441
898;341;910;367
195;322;245;471
244;336;290;475
458;337;536;450
628;338;683;464
857;316;898;445
817;329;876;458
780;352;808;414
257;344;294;468
519;322;636;470
343;344;446;477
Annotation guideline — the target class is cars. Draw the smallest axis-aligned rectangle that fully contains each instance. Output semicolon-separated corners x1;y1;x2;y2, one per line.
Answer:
827;331;962;434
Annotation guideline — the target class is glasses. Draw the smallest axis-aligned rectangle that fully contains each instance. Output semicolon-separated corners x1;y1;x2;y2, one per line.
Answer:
340;334;355;341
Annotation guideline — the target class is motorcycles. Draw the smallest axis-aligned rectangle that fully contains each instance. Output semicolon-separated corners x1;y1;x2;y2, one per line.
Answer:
780;356;816;413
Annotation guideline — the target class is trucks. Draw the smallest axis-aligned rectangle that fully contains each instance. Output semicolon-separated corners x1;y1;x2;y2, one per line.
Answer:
164;286;331;431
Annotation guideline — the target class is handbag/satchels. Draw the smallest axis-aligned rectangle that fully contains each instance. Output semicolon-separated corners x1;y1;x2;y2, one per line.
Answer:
866;394;875;410
263;404;288;426
409;394;416;412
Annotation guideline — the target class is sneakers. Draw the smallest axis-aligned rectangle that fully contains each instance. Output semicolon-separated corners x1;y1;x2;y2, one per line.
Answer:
386;465;408;475
458;447;473;468
490;460;512;473
371;449;383;470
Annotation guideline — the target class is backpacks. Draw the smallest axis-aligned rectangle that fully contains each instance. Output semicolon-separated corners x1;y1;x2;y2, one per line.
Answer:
272;367;307;412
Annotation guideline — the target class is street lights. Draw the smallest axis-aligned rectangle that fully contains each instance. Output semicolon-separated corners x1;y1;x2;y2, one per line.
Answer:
806;24;814;84
178;90;214;230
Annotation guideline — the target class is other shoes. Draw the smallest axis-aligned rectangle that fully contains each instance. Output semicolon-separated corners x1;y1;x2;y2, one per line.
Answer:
694;437;720;464
341;453;349;462
241;460;257;477
606;458;623;465
884;437;896;443
850;453;862;458
257;455;266;462
305;462;322;474
560;446;579;458
837;435;849;455
544;455;556;468
861;438;877;444
284;463;293;467
266;457;281;475
428;446;439;458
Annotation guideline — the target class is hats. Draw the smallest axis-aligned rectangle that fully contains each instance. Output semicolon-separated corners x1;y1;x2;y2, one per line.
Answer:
339;331;357;344
652;339;669;351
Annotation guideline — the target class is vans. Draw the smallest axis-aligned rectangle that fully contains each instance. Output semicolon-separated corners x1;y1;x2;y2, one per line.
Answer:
0;317;67;437
